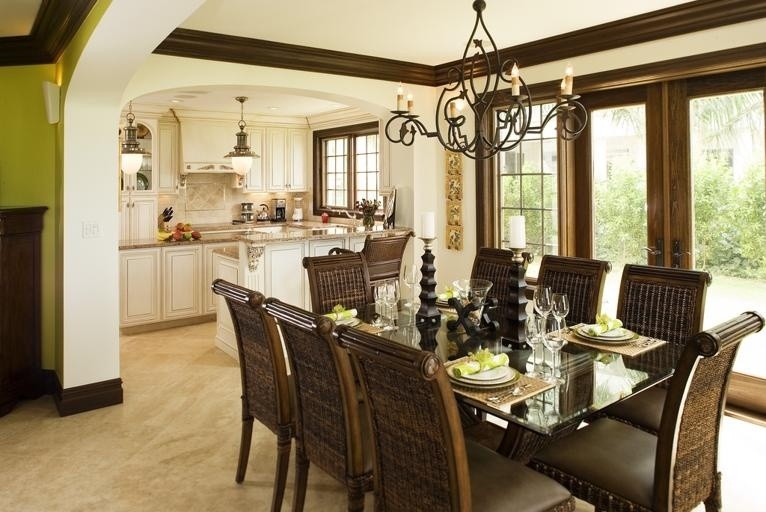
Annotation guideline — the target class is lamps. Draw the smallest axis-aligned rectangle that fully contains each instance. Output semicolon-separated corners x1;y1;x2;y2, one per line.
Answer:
384;0;590;161
223;96;260;177
40;80;60;126
120;99;147;176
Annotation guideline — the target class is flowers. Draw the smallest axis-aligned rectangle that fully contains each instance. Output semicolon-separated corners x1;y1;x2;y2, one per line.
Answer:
354;197;382;227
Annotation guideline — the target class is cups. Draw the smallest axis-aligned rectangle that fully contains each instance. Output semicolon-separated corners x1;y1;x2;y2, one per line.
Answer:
454;278;494;327
322;215;328;223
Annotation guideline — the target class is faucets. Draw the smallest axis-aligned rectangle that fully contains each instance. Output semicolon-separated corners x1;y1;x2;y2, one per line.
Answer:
339;210;357;220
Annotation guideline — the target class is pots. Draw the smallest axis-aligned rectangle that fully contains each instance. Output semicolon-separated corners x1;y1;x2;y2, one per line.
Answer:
240;212;254;223
240;202;253;212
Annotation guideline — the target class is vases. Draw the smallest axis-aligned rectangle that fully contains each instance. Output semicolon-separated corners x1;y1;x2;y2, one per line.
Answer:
363;213;375;230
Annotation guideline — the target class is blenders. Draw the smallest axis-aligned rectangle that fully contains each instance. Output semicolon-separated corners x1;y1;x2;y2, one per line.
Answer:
291;197;304;221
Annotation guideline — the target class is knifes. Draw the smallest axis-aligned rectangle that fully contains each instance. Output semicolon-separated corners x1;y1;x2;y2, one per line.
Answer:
163;207;174;222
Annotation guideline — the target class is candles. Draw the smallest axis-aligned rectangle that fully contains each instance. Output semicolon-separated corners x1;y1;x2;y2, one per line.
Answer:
509;214;526;248
421;212;437;239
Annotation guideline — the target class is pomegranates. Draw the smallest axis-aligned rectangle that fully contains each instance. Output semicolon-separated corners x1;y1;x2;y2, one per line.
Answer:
176;223;184;231
184;225;192;232
192;231;201;238
173;232;183;240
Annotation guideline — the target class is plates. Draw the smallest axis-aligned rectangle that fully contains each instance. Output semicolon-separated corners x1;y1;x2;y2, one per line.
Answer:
446;361;522;389
436;291;459;308
137;173;149;190
136;124;149;139
334;317;364;328
574;324;640;345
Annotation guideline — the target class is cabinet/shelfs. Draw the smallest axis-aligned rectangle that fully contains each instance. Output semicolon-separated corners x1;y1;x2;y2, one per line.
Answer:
118;194;157;246
265;126;312;194
236;126;265;194
119;115;158;193
159;117;180;195
119;244;203;337
0;206;49;417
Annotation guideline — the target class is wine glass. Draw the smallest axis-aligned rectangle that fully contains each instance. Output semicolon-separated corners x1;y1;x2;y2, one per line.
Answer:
374;264;420;330
523;285;570;386
525;386;563;427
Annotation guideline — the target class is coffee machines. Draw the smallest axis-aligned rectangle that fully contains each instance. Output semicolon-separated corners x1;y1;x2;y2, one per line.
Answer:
271;199;286;221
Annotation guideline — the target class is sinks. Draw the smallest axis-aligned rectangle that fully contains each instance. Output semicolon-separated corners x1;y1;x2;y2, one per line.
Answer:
328;223;348;227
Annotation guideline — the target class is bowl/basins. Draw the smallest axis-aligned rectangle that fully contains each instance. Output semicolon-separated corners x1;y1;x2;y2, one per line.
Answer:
182;231;194;240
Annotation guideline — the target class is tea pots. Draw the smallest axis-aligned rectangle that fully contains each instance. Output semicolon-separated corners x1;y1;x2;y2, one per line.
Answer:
256;204;269;220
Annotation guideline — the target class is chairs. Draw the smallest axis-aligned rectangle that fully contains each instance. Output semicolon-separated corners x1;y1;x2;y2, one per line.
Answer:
210;228;765;512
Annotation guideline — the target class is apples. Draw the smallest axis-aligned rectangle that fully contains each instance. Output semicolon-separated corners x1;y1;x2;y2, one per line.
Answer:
184;233;191;239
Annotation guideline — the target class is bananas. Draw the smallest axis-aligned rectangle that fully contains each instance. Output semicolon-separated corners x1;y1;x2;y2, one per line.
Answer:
156;227;173;240
